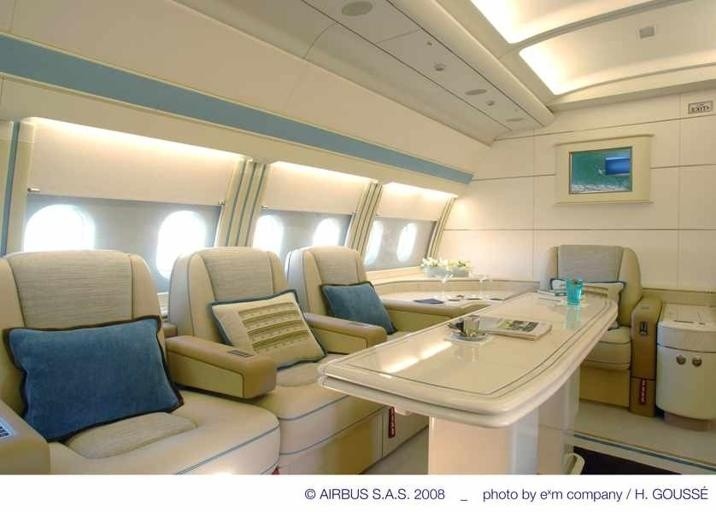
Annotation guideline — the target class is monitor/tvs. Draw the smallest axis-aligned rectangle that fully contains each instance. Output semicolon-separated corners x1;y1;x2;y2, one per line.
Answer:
568;146;632;193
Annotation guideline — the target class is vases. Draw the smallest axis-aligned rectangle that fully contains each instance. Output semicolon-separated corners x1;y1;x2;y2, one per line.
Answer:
425;267;468;280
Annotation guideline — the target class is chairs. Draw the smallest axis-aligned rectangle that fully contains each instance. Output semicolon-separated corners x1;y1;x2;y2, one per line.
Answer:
536;244;662;417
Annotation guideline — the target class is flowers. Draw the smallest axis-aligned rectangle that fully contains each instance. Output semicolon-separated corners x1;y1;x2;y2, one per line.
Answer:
419;258;471;270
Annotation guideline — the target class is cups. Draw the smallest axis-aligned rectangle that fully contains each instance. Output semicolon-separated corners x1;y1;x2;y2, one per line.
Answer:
565;275;582;305
462;317;480;336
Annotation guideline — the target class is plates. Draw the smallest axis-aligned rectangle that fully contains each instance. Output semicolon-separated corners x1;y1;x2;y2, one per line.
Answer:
455;331;488;341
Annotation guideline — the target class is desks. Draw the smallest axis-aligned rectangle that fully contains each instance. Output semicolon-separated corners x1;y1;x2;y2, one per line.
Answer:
320;291;621;475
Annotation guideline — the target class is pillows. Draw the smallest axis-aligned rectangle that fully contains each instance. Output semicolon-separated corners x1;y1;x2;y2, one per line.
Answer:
209;289;326;374
5;316;183;439
549;278;626;331
319;281;398;336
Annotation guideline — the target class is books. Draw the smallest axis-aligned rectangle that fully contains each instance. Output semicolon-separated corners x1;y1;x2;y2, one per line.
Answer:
415;287;504;304
477;311;553;340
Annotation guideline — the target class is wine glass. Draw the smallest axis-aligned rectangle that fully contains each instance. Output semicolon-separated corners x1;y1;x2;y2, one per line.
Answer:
471;269;489;298
433;271;452;300
